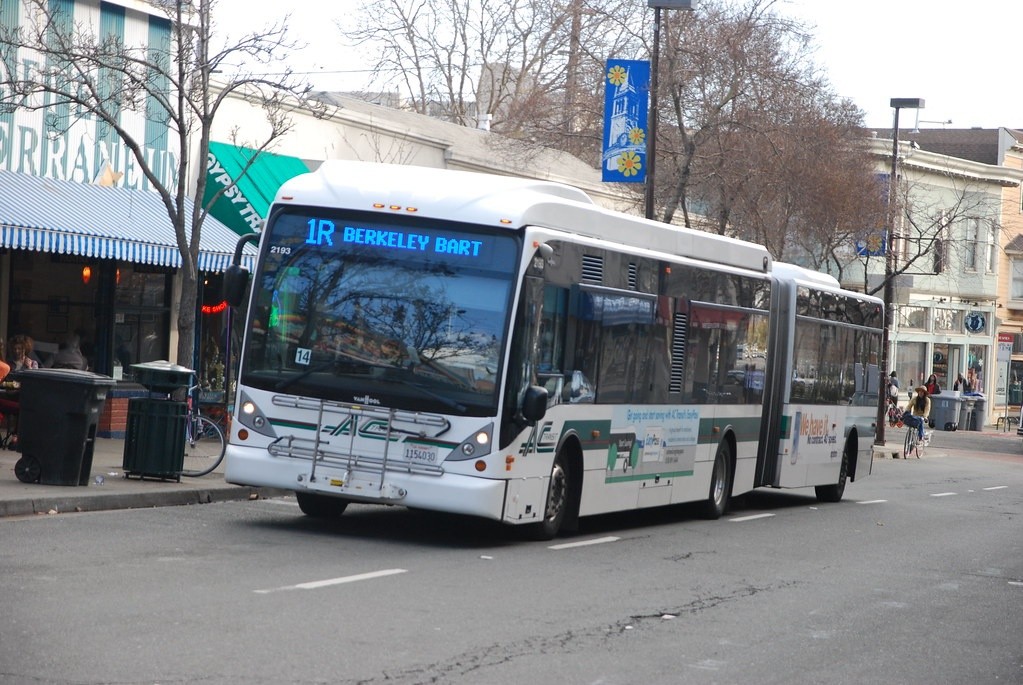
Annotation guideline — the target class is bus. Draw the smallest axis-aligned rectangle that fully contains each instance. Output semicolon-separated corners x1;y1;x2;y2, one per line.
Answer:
223;158;886;543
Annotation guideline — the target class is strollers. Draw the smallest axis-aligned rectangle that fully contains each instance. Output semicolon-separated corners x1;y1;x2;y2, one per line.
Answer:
887;395;905;428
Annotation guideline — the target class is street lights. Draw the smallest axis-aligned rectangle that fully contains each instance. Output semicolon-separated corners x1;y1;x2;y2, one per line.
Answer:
874;98;927;447
646;0;698;220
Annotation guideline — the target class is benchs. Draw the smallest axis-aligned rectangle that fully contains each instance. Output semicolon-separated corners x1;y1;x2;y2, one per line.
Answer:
995;406;1023;432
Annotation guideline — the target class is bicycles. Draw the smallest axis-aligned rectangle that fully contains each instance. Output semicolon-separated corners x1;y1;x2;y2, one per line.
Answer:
902;417;932;459
183;382;227;478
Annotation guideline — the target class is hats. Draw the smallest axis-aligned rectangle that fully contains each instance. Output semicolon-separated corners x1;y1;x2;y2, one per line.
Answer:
915;385;927;395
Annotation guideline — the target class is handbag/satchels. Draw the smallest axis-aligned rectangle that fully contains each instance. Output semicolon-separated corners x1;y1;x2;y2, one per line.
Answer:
890;384;899;397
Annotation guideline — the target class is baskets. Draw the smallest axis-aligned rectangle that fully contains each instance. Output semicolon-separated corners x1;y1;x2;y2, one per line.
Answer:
904;417;921;429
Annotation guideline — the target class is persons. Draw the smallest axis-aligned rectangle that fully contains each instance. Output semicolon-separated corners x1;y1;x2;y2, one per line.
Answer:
954;372;983;393
924;374;941;394
890;371;901;406
905;386;931;444
0;332;159;409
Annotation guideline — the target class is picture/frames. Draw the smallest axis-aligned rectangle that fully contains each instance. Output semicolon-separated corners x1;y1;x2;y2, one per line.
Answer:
46;294;69;334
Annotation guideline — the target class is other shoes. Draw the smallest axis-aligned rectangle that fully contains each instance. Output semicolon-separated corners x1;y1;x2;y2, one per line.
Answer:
917;437;923;445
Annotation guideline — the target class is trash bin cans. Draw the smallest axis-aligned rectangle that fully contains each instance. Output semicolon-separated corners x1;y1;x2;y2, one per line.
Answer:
123;361;198;482
930;389;988;432
11;367;117;487
